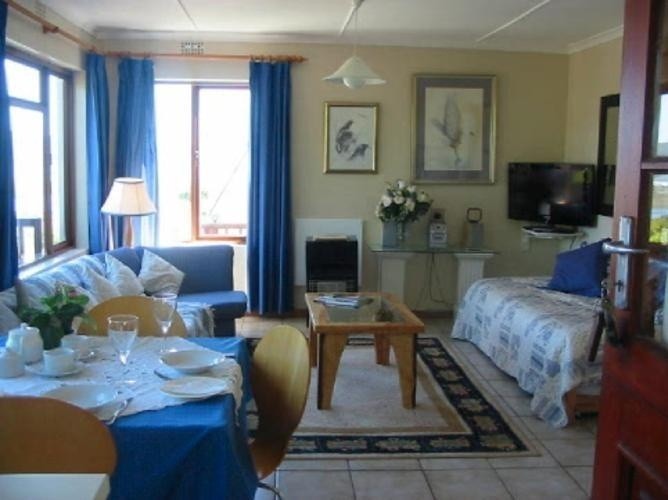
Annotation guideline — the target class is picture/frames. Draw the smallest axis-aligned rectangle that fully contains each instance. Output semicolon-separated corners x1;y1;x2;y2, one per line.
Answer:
321;100;380;174
409;72;496;186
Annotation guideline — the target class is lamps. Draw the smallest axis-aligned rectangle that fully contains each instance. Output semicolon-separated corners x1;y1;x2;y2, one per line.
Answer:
98;177;158;248
318;5;387;91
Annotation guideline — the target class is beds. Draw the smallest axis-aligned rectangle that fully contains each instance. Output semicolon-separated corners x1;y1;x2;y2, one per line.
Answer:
448;261;667;431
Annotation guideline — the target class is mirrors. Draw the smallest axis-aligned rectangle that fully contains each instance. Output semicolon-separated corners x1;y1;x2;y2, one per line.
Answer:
595;82;666;220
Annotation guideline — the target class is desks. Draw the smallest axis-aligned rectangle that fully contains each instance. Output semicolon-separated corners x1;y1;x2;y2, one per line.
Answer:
0;472;112;500
365;242;503;319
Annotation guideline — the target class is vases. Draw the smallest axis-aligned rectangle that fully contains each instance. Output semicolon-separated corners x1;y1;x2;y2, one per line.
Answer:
393;222;413;248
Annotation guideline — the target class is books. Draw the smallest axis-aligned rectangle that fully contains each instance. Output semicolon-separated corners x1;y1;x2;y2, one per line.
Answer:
313;291;373;311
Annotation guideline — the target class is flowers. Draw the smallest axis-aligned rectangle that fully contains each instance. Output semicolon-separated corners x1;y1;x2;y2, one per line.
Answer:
374;178;433;225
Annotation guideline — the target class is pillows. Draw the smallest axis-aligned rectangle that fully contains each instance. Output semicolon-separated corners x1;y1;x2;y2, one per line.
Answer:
104;252;144;296
537;237;610;299
79;262;122;304
12;275;44;321
136;249;186;299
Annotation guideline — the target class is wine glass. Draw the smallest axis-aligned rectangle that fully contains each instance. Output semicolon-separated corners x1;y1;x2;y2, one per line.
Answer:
107;313;139;386
151;293;179;356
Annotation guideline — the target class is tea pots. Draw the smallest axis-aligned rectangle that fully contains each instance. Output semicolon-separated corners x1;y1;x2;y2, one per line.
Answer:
0;344;27;376
5;322;43;364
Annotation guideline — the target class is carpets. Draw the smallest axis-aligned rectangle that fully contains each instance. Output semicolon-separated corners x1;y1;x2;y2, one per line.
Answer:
281;334;541;458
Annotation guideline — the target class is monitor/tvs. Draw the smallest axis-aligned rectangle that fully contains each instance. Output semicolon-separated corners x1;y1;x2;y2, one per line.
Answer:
507;162;596;234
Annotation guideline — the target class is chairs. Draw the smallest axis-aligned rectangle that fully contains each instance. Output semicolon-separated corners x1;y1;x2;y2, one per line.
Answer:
247;324;314;499
0;396;118;481
76;295;190;338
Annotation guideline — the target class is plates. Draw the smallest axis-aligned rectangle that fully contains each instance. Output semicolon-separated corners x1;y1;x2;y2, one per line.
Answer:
26;363;89;379
45;384;114;412
160;350;226;373
159;376;225;398
76;346;99;361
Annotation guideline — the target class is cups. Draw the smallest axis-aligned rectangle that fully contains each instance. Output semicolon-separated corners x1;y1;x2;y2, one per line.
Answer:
42;348;74;374
61;333;89;356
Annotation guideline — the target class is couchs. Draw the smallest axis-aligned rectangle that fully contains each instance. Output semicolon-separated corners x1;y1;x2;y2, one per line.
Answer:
0;241;247;338
